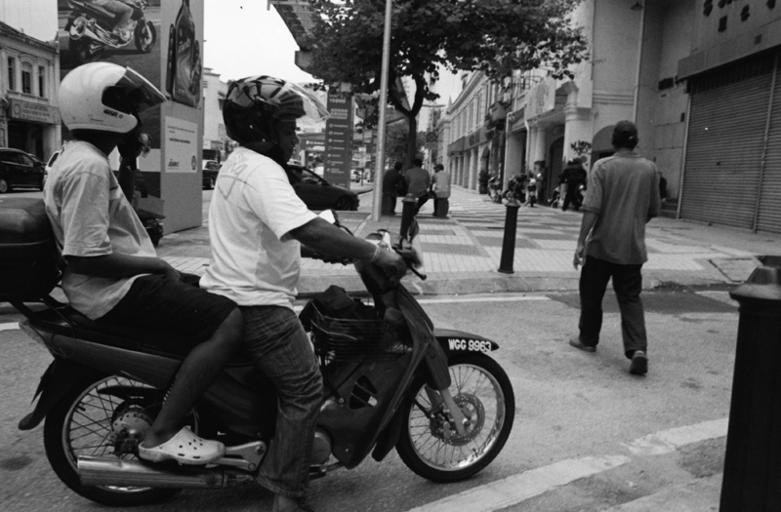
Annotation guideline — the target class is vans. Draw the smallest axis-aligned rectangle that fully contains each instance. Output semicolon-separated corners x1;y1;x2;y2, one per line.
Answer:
42;149;63;195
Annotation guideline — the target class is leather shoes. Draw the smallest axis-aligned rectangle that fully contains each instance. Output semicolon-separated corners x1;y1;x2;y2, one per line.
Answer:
628;350;647;375
569;337;595;353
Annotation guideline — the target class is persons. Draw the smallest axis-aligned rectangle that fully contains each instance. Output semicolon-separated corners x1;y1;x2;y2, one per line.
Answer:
568;120;660;375
414;163;451;216
559;158;587;211
43;61;247;465
383;162;408;213
198;75;408;512
91;0;134;43
404;158;430;196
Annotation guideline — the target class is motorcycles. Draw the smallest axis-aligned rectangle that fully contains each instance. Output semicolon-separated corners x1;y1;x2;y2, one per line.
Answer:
0;192;516;509
521;175;544;208
503;177;525;202
488;175;502;204
561;179;586;211
64;1;157;66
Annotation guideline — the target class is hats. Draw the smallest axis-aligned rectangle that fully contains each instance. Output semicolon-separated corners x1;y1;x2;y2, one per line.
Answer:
613;120;637;142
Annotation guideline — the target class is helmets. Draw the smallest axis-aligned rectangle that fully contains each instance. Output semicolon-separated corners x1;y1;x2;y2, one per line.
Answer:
57;59;166;135
221;74;330;143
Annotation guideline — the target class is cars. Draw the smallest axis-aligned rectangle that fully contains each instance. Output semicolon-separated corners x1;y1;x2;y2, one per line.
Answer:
283;164;360;212
302;166;367;184
0;147;46;194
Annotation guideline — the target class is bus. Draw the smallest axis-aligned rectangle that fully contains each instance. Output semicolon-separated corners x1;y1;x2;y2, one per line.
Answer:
201;160;221;190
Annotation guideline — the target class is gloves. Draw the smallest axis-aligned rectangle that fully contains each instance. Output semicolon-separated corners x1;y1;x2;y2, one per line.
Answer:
370;245;406;279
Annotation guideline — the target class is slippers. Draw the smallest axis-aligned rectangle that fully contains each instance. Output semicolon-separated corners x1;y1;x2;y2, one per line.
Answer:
137;424;225;466
